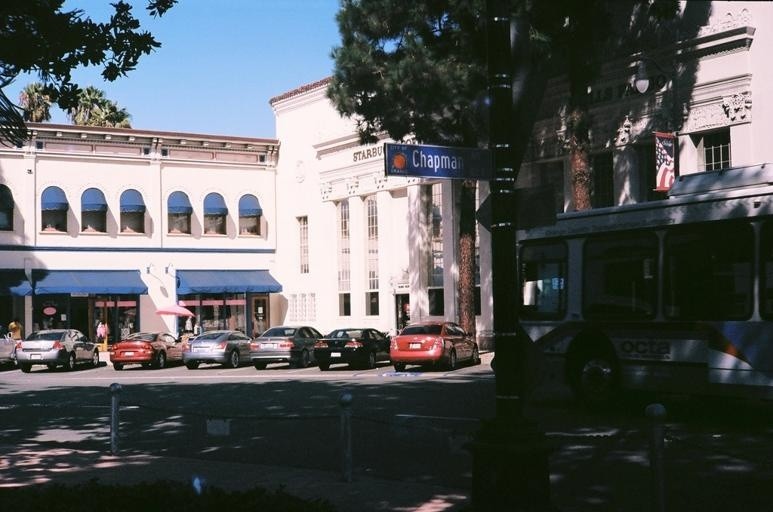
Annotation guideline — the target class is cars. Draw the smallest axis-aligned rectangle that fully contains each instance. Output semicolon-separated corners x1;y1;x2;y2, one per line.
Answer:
12;327;99;370
249;324;322;370
313;328;390;370
178;330;253;369
109;331;182;369
388;319;480;374
0;327;19;368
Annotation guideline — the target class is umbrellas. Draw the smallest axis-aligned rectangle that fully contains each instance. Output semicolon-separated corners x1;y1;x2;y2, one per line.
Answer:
156;304;197;319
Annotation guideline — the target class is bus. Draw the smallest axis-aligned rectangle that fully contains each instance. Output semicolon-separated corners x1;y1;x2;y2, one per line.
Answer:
506;160;771;411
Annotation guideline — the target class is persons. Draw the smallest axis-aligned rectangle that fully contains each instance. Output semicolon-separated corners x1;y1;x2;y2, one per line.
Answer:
185;316;195;331
8;318;23;340
96;321;105;338
403;302;411;330
256;319;263;333
